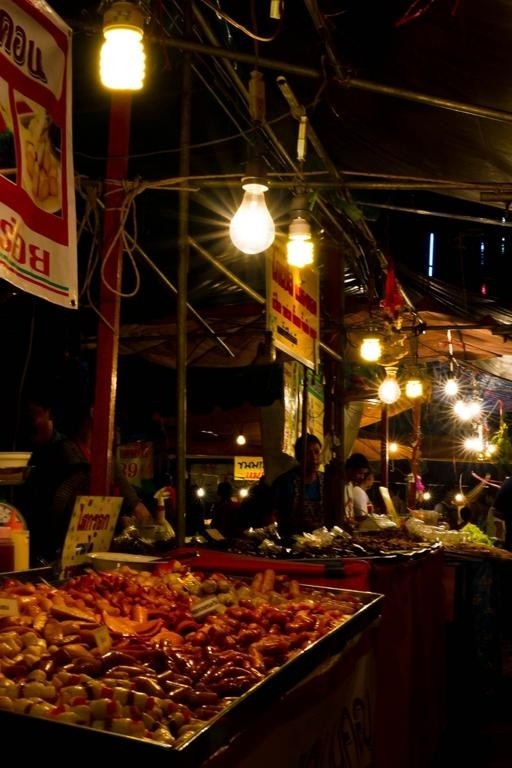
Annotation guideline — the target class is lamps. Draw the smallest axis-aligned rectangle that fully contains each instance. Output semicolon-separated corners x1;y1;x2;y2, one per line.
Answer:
286;187;314;269
229;1;276;256
97;0;147;91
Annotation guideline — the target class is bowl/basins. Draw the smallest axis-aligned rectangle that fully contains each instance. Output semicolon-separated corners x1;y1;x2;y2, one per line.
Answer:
85;551;173;575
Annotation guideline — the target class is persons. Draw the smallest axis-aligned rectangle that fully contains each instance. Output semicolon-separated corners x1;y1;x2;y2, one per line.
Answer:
18;386;66;482
151;469;269;539
268;433;346;546
14;367;156;567
326;452;512;552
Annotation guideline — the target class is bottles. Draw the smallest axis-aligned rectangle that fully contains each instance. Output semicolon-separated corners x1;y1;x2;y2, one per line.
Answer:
0;525;30;572
